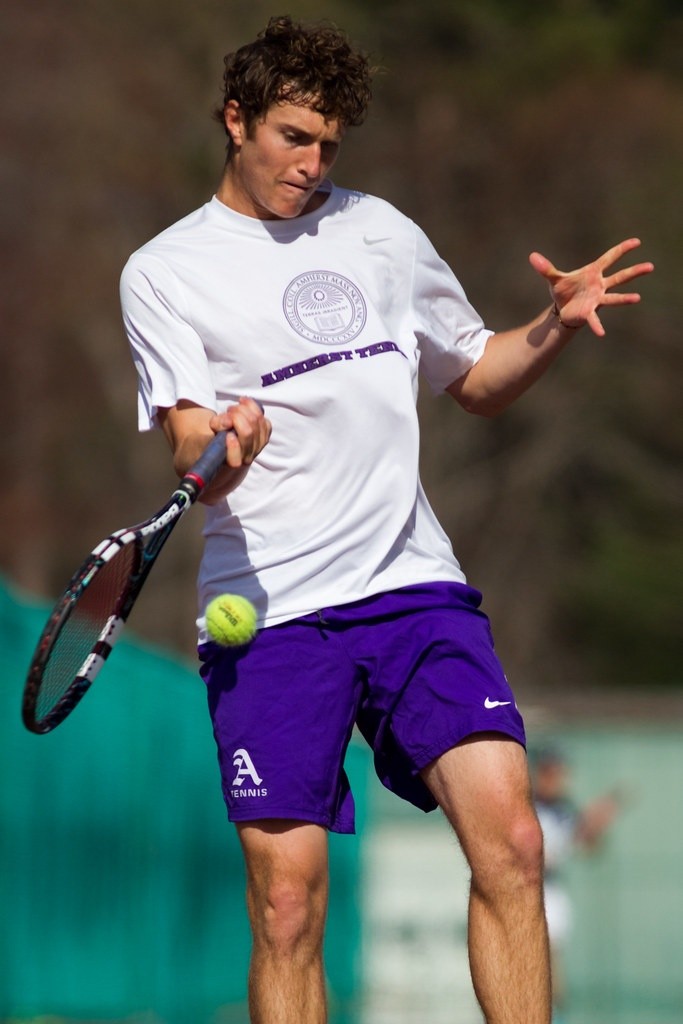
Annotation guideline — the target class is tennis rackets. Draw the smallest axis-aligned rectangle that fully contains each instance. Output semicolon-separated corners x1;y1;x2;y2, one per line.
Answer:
20;400;265;737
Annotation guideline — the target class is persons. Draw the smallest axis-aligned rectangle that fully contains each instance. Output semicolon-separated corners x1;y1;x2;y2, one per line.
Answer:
119;16;657;1023
527;743;632;1008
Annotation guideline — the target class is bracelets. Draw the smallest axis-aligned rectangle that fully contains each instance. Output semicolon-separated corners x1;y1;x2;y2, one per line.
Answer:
553;302;581;330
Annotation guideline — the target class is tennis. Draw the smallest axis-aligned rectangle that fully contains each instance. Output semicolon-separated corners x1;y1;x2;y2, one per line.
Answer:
205;593;258;645
